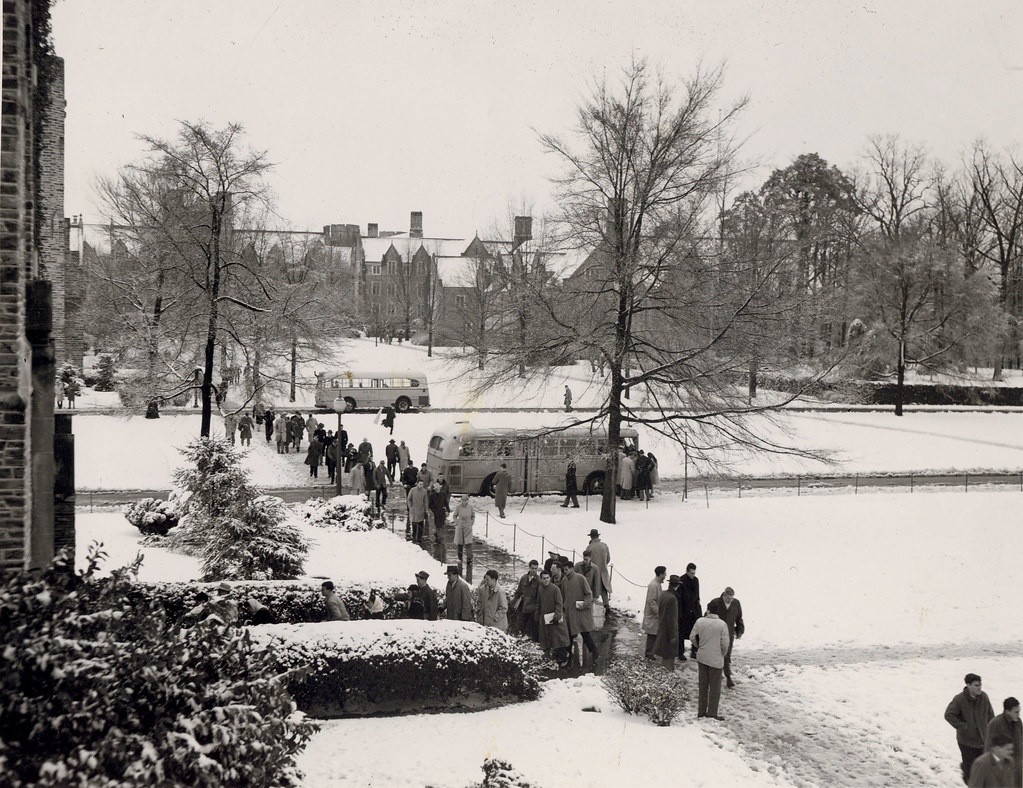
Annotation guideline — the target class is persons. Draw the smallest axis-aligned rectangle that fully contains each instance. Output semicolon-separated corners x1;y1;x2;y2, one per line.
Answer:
365;594;384;619
195;592;209;606
617;444;659;501
244;599;277;625
942;672;995;786
217;363;242;406
516;548;602;664
642;563;703;670
253;401;475;562
224;412;254;446
441;565;473;619
690;587;745;722
54;379;75;409
322;581;350;621
585;529;610;613
564;385;574;413
478;570;509;630
491;463;513;519
381;403;396;435
968;736;1016;788
559;453;581;508
217;582;232;595
985;697;1023;788
393;570;439;621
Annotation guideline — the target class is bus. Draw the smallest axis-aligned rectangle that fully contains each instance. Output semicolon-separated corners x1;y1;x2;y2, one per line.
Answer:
314;369;430;413
426;428;639;500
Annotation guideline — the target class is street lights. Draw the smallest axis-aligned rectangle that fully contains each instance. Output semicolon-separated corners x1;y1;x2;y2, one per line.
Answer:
333;388;347;496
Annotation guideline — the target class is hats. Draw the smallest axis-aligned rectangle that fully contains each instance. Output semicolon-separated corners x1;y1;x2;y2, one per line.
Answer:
666;575;682;583
217;583;231;591
416;571;429;579
587;529;601;535
443;566;458;574
389;440;395;443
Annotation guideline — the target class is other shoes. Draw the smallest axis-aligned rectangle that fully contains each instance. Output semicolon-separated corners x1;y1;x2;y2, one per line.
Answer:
646;655;655;661
678;655;686;661
690;653;696;659
560;504;566;507
726;679;733;686
592;648;598;659
712;716;725;721
571;506;578;508
561;652;569;667
